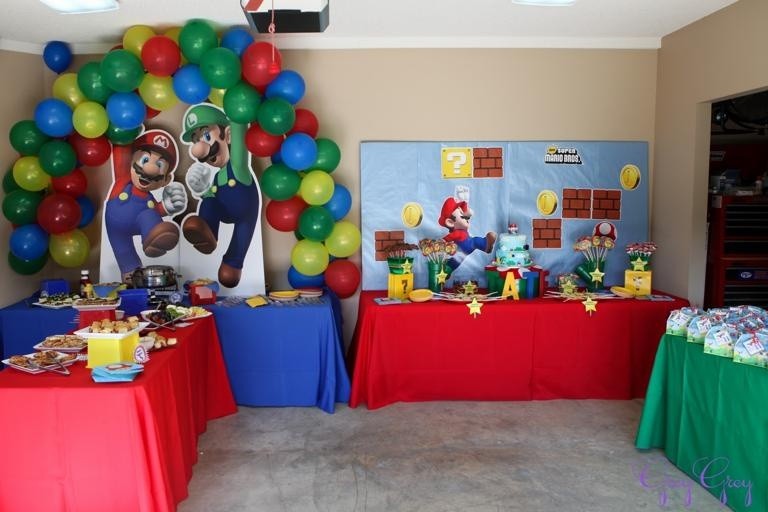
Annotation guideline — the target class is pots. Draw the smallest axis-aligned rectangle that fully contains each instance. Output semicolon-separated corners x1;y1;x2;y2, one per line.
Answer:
128;266;182;289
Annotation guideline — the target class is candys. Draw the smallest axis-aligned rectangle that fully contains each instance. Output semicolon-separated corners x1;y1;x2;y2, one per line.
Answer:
623;241;659;269
572;236;616;263
418;237;458;268
384;243;420;261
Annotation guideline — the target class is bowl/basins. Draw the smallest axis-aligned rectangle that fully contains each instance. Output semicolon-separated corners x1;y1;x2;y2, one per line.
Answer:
73;317;151;341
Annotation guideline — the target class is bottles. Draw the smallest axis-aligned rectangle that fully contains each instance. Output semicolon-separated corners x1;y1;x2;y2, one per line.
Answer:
78;270;93;297
754;176;763;192
713;186;718;195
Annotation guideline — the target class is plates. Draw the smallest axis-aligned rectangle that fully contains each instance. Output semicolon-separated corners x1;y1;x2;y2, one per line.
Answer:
2;350;76;375
171;304;213;321
31;293;78;311
73;299;123;310
408;289;432;302
142;311;187;331
268;286;324;302
34;332;89;351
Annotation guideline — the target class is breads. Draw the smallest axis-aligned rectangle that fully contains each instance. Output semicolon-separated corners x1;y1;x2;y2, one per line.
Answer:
90;316;142;334
10;350;77;370
148;331;178;350
43;333;87;350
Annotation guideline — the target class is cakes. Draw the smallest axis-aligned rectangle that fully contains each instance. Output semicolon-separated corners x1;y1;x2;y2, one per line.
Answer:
493;223;533;267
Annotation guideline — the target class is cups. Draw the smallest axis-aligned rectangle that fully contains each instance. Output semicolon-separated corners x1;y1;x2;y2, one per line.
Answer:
116;310;125;320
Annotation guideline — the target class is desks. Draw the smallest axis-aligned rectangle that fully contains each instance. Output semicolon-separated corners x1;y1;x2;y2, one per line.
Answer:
635;331;768;512
0;308;238;512
0;284;351;414
347;287;690;410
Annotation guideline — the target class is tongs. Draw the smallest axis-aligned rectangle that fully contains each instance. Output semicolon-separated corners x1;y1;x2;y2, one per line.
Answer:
144;311;176;333
27;351;69;377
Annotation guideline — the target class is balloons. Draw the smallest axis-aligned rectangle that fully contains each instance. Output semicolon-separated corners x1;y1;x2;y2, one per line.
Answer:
1;17;362;300
